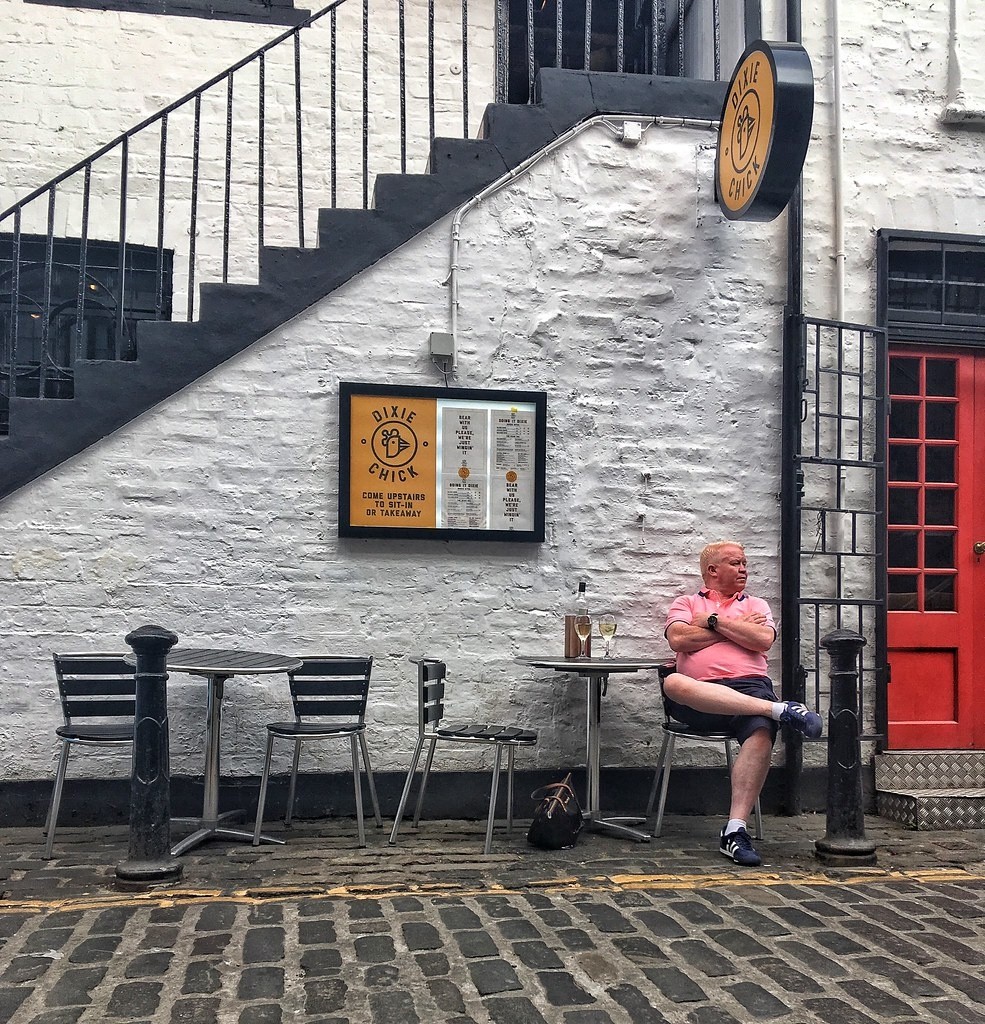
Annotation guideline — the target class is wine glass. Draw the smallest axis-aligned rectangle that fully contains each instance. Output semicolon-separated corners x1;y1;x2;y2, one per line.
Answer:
574;615;593;660
599;615;617;660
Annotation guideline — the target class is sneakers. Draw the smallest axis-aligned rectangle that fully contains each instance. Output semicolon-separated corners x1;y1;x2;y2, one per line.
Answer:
718;826;761;865
779;701;822;738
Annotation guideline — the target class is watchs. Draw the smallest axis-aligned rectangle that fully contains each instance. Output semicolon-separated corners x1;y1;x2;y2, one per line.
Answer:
707;613;718;629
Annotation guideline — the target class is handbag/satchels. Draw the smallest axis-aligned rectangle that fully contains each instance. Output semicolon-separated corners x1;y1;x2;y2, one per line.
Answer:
526;773;584;850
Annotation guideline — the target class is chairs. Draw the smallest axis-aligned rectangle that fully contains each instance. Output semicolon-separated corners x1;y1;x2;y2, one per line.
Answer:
251;655;383;848
390;657;538;854
646;658;764;840
40;652;136;859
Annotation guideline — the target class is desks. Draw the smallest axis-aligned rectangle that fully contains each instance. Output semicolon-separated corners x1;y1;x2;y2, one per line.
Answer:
514;655;675;843
123;648;304;857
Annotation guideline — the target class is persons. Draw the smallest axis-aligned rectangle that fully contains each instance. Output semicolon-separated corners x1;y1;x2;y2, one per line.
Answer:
663;540;823;867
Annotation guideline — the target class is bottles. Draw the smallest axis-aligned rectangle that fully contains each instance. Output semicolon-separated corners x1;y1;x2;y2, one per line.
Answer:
572;582;588;615
